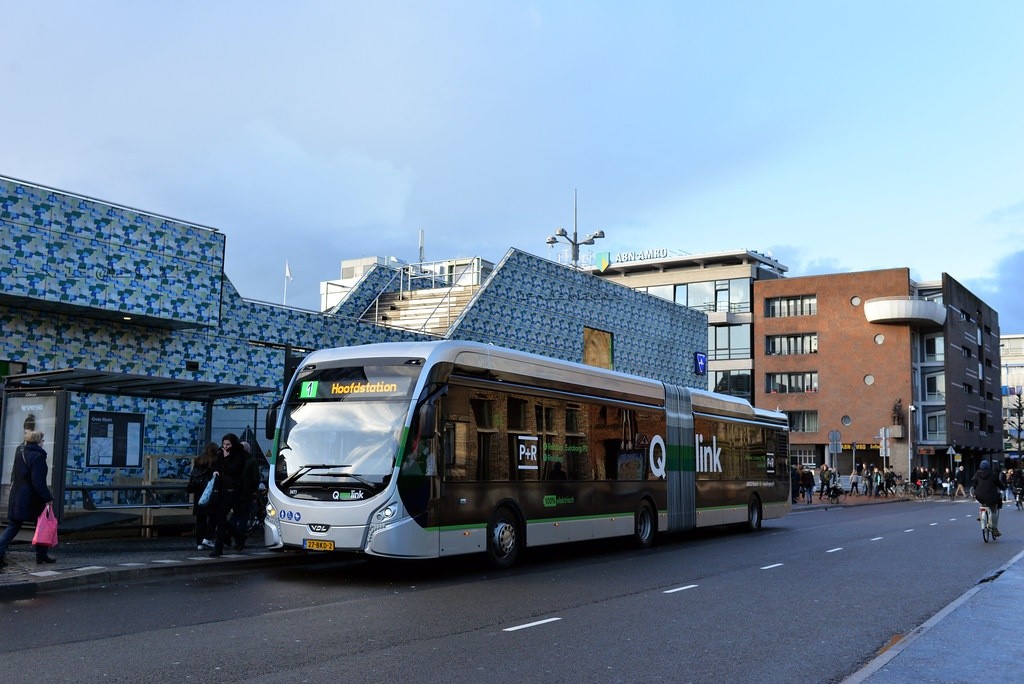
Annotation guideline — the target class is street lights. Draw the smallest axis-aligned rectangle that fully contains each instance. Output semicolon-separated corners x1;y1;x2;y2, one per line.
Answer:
546;188;605;272
907;403;917;494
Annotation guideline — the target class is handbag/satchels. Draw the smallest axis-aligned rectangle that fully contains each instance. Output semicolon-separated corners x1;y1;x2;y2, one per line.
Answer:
198;475;216;506
32;504;58;547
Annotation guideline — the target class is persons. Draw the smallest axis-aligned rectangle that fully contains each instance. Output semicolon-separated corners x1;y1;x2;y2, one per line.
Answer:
911;467;938;500
861;463;902;498
791;463;815;503
971;461;1005;536
849;469;859;496
548;461;567;479
1000;468;1024;505
818;464;841;499
955;466;969;498
0;427;57;567
190;434;260;557
941;468;954;498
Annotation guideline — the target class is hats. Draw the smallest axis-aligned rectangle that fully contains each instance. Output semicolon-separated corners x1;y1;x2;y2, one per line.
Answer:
980;461;988;469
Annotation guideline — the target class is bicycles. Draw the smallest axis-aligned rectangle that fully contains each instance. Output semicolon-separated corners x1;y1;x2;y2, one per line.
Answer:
1015;487;1023;513
887;477;957;504
976;496;1006;543
829;482;847;504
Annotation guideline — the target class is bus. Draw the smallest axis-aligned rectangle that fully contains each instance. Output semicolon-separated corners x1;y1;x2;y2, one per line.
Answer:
262;339;793;571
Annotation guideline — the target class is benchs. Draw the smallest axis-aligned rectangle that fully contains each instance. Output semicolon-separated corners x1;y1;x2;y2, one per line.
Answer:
63;453;198;504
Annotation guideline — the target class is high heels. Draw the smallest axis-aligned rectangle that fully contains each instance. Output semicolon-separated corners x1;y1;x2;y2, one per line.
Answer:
37;556;56;564
0;562;9;568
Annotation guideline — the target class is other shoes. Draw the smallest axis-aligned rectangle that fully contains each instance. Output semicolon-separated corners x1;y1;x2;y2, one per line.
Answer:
198;545;207;550
978;513;980;520
202;539;215;548
209;549;222;557
993;529;1000;535
236;534;246;551
